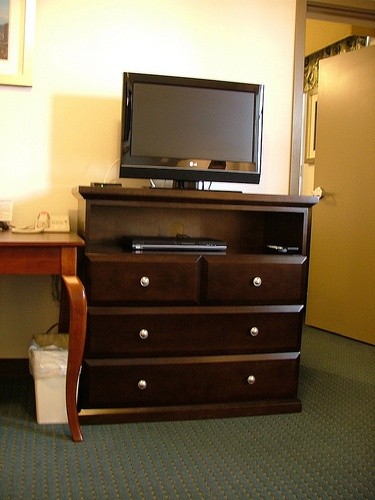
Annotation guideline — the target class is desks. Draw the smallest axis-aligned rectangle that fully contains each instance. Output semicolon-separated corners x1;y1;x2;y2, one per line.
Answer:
0;230;86;442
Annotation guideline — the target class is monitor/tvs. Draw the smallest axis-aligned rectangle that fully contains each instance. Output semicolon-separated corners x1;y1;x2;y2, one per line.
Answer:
119;72;265;192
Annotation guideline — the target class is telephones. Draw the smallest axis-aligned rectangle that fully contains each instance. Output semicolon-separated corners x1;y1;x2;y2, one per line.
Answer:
35;210;71;233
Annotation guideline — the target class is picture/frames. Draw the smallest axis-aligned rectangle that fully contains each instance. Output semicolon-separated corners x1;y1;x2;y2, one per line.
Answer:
304;87;318;163
0;0;36;87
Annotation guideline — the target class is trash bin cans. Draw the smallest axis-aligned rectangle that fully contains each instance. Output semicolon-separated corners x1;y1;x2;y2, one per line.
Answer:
32;332;81;425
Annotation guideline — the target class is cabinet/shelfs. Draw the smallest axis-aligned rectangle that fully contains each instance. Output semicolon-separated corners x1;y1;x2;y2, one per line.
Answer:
78;186;317;426
